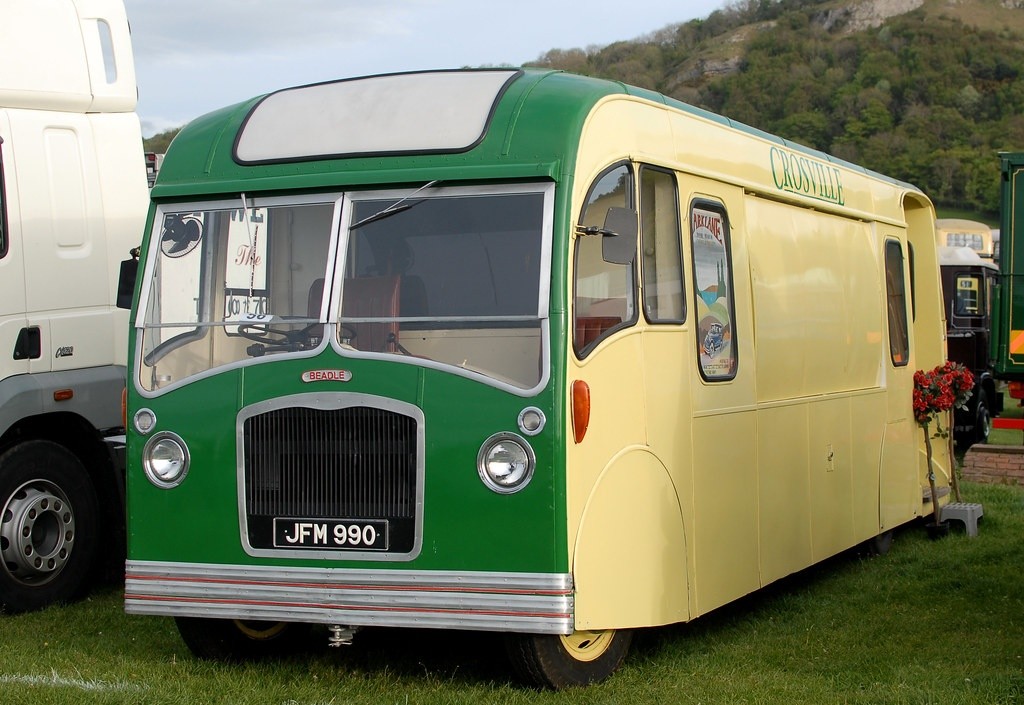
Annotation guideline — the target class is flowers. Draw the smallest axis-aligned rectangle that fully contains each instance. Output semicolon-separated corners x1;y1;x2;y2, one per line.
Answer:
913;360;976;443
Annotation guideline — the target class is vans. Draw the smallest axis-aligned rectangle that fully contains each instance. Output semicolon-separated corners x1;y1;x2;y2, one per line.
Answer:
1;0;162;615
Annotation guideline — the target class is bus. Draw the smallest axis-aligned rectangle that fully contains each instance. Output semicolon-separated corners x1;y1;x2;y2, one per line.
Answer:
116;65;956;694
935;218;1001;452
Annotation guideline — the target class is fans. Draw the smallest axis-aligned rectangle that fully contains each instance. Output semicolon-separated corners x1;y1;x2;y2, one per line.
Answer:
161;217;204;259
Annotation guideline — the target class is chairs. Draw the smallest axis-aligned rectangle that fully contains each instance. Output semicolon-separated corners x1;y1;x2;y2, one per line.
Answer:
538;316;622;382
307;273;402;353
